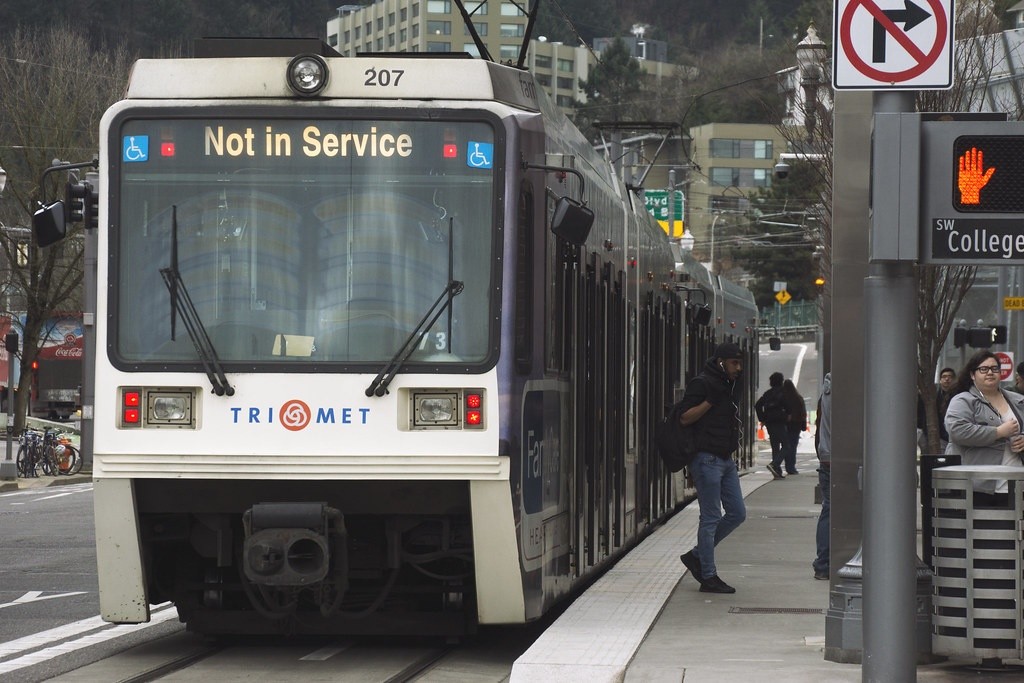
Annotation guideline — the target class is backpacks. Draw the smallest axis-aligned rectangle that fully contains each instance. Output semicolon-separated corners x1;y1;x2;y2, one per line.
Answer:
656;374;710;473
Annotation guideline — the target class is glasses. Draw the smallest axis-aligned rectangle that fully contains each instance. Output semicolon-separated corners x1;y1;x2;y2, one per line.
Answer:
975;365;1000;373
940;375;953;378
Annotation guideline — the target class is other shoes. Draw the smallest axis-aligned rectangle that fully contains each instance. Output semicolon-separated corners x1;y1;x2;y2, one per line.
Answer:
700;576;736;593
767;463;780;477
813;569;829;580
772;475;786;480
681;549;703;583
788;470;798;475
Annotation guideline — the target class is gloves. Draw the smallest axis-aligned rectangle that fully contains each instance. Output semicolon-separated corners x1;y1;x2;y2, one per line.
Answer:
705;388;720;405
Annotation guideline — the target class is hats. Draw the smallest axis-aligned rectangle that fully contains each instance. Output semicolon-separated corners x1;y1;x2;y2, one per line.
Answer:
703;343;743;362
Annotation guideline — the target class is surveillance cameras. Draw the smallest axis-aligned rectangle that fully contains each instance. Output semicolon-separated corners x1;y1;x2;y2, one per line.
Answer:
775;164;789;178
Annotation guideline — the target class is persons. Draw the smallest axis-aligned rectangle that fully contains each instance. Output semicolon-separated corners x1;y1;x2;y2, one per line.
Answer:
936;368;956;442
944;350;1024;509
812;371;832;580
1005;361;1024;396
755;372;791;480
679;341;747;593
784;380;806;474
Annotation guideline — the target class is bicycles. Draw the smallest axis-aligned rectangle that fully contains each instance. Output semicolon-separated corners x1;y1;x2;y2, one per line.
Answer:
16;422;83;479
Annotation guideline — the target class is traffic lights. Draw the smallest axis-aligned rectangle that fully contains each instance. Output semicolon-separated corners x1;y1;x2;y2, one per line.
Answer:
954;135;1024;214
63;181;84;224
84;179;99;230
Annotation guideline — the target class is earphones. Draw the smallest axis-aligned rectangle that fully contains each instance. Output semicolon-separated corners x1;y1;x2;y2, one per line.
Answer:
720;362;725;370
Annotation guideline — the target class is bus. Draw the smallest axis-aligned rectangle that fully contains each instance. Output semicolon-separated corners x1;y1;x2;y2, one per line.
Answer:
32;2;781;640
